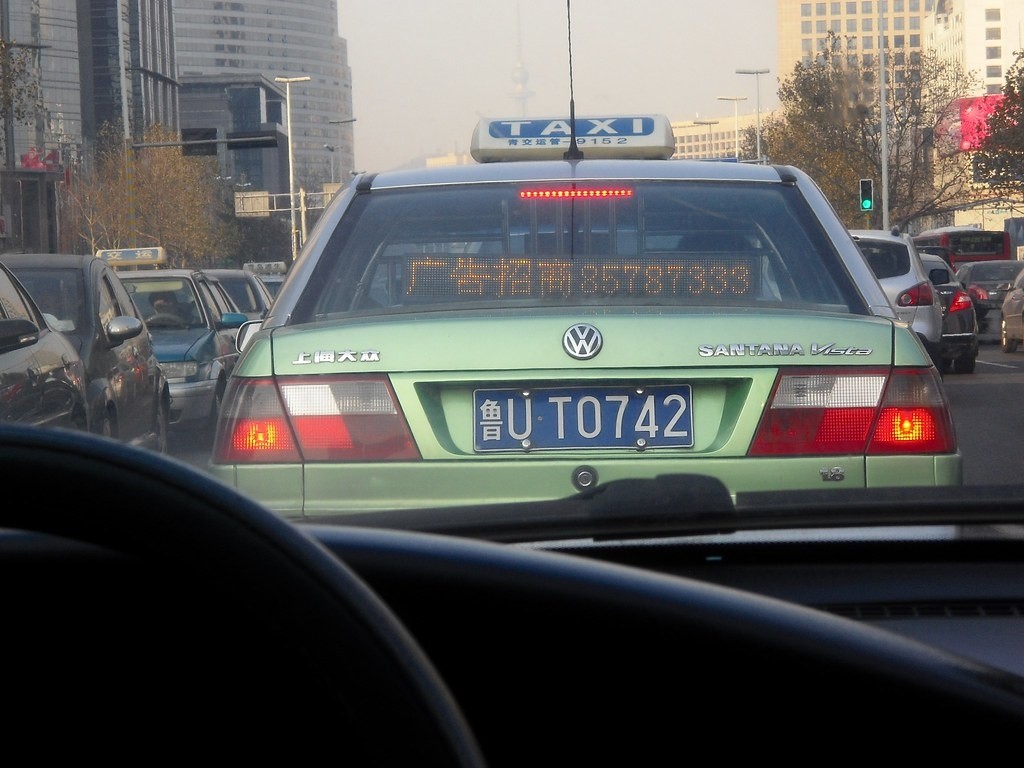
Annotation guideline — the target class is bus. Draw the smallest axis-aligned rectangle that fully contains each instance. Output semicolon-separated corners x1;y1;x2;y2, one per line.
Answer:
911;226;1011;275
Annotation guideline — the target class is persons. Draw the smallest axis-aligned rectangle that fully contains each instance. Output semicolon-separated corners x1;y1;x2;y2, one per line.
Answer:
148;289;195;326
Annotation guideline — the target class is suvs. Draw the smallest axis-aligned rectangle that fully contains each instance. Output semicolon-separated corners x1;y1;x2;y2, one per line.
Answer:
846;227;943;363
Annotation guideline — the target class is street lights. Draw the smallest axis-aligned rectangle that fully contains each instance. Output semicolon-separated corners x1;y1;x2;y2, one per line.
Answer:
348;170;366;177
736;67;770;165
718;96;748;161
275;77;310;260
693;120;719;159
323;143;343;183
329;118;357;182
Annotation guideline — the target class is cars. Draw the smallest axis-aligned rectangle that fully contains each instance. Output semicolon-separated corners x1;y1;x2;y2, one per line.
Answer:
243;261;288;303
199;269;273;324
954;260;1024;340
0;259;89;435
919;252;978;374
210;116;964;518
0;254;170;455
95;247;248;450
997;268;1024;353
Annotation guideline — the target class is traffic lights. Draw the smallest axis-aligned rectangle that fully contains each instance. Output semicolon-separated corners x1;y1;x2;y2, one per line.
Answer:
859;179;873;211
